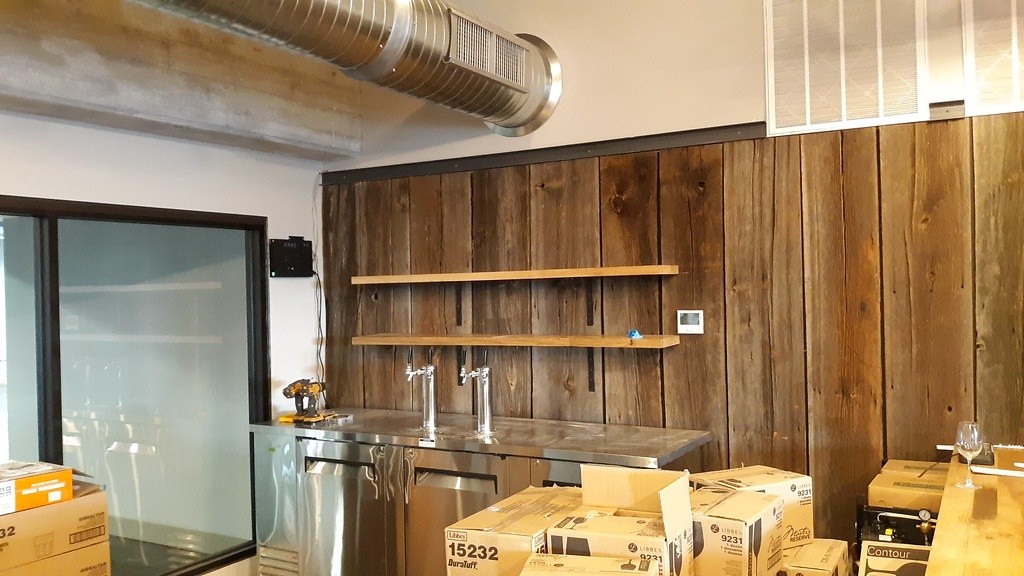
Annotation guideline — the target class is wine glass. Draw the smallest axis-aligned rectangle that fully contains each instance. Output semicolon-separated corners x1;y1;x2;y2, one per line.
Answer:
955;420;983;489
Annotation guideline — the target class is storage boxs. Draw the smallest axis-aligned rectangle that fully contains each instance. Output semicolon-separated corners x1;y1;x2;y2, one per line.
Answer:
444;457;950;576
0;462;112;576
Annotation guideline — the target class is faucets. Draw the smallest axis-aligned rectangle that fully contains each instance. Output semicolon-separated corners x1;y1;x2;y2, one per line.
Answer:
459;367;492;434
404;365;436;430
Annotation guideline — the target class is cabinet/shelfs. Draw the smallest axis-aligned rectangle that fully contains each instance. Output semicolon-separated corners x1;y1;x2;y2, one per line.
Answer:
352;264;680;350
